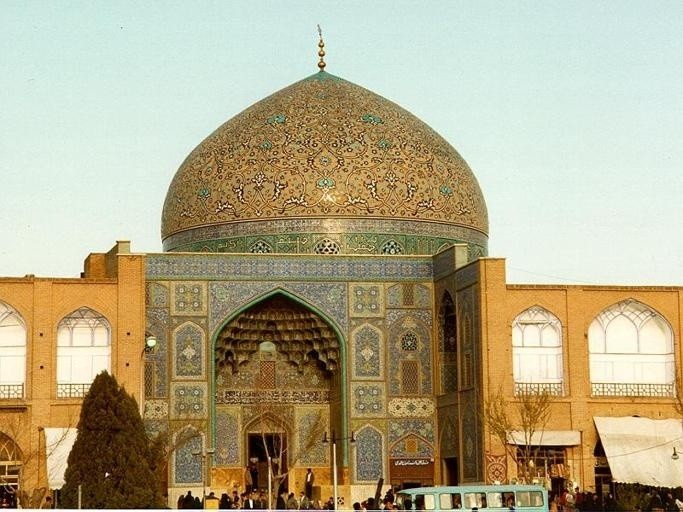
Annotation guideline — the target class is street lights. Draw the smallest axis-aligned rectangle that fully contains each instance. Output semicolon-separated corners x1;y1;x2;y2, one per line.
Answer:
321;429;358;512
190;448;216;510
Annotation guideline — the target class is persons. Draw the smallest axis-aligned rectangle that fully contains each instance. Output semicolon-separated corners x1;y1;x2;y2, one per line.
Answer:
305;468;314;500
177;489;335;511
549;486;616;512
452;494;461;509
41;496;52;509
354;494;423;511
643;487;683;512
244;465;259;493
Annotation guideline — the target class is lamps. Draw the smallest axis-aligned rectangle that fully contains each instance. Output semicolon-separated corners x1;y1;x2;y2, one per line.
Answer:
144;335;157;352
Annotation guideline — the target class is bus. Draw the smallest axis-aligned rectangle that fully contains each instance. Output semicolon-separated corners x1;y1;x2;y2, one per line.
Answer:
392;482;550;511
